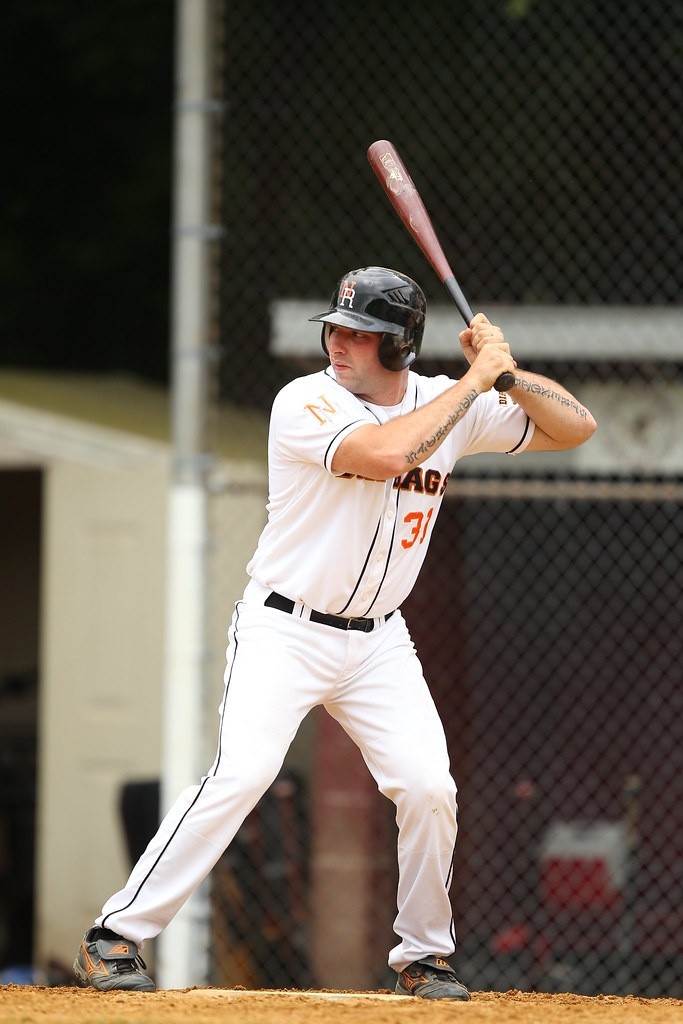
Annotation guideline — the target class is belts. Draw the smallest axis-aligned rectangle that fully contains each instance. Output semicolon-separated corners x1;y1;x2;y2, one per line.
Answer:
264;592;395;633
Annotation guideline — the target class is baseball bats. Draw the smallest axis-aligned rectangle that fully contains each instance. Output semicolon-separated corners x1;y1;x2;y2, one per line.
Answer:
366;137;517;394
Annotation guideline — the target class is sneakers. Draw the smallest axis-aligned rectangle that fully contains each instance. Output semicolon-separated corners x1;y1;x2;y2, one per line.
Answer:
72;926;156;992
397;955;471;1003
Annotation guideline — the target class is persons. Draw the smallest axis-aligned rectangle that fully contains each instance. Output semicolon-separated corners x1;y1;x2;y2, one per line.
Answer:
74;266;595;997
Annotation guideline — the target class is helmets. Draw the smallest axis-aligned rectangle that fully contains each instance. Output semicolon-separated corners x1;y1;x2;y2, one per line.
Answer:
308;265;426;372
